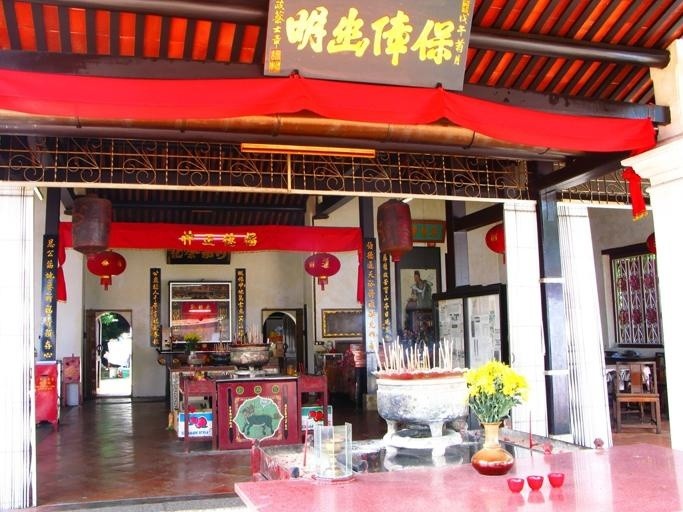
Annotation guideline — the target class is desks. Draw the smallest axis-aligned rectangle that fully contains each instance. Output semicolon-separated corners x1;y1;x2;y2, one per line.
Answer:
225;426;683;512
175;370;329;454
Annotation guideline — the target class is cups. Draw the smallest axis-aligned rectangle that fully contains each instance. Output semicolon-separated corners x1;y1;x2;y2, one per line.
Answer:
527;475;544;491
548;472;565;488
508;478;524;493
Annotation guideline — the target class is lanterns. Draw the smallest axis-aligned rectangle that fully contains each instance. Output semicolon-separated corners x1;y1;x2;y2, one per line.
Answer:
646;232;656;254
86;251;126;290
485;223;506;265
304;253;340;290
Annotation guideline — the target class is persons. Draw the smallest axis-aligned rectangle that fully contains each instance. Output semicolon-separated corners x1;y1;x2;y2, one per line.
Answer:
409;271;433;306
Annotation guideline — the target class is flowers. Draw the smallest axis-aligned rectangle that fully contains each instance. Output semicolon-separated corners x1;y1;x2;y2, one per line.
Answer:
460;358;528;422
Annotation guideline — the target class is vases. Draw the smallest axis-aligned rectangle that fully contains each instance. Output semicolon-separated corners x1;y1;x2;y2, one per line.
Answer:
470;419;515;477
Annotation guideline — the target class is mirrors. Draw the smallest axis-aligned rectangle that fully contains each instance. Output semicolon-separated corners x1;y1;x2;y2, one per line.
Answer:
169;280;234;342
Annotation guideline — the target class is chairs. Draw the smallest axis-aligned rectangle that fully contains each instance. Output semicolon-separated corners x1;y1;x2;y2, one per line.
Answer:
611;359;664;436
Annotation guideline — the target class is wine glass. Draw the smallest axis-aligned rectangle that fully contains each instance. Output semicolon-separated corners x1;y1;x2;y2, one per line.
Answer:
321;437;345;478
